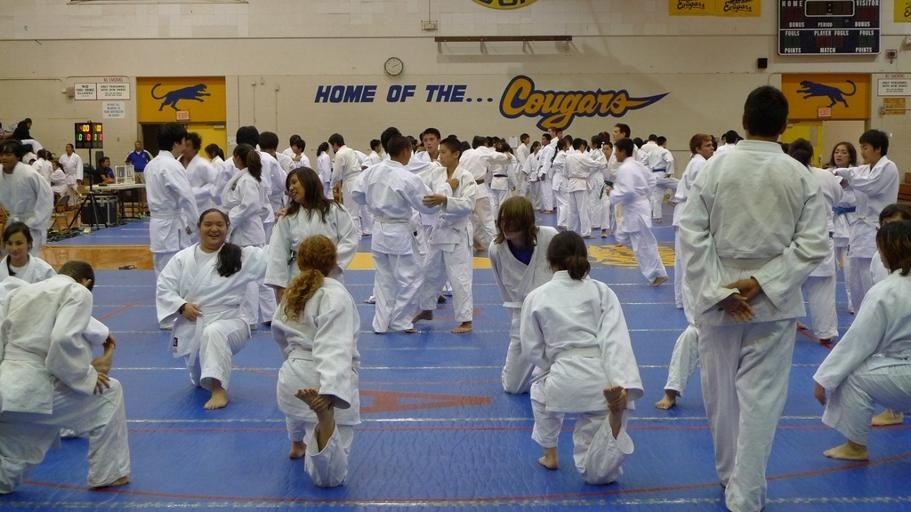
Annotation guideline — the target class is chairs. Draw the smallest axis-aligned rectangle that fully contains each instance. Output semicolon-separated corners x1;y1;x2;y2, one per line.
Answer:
51;195;70;232
70;186;90;225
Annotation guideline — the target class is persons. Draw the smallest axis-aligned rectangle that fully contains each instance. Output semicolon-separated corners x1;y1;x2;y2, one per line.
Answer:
810;127;911;461
94;157;114;185
672;133;714;310
784;136;845;349
124;140;153;219
143;120;681;488
678;86;827;512
0;260;130;495
105;156;114;178
697;130;790;159
0;221;55;284
653;326;700;409
0;136;54;259
0;275;116;438
14;116;83;197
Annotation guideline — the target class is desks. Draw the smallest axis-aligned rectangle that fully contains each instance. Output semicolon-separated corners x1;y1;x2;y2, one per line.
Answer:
87;183;145;227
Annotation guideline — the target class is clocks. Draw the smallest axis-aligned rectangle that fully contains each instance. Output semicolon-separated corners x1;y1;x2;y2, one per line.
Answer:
385;57;404;79
